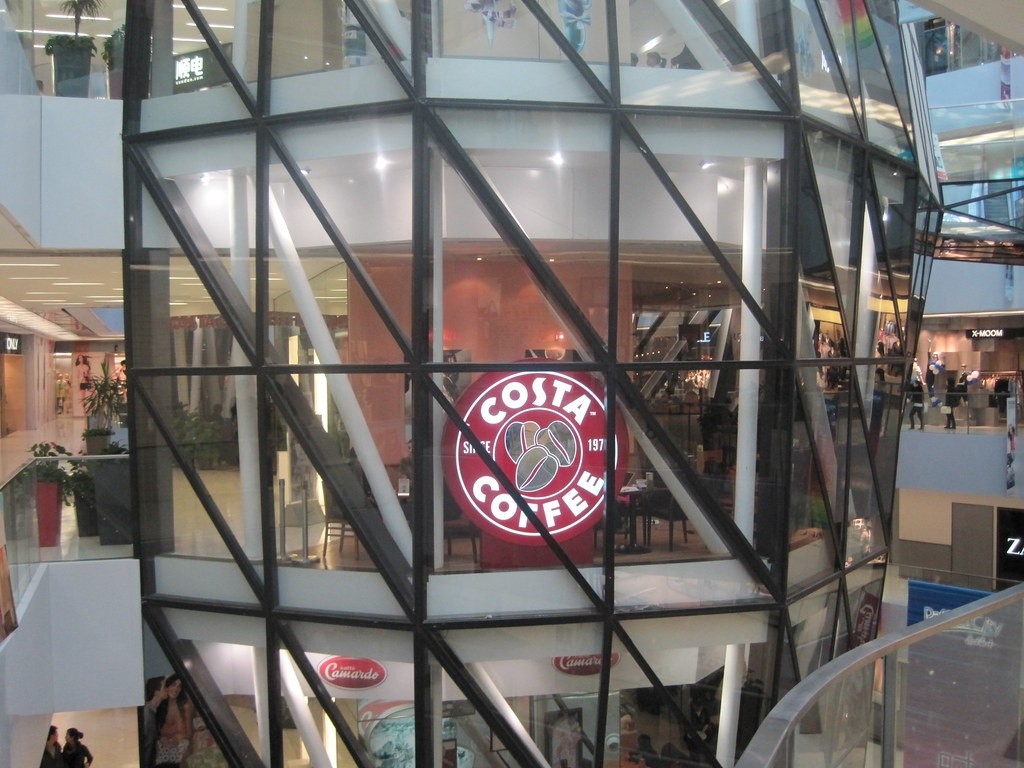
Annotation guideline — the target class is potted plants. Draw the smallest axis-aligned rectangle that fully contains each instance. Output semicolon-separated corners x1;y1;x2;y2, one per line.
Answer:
86;440;135;545
45;32;97;98
81;428;116;456
80;361;126;431
102;23;129;102
168;401;223;471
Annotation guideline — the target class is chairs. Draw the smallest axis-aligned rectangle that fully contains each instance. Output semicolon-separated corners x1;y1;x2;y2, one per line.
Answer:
641;488;690;553
322;481;360;560
444;515;481;564
594;500;628;549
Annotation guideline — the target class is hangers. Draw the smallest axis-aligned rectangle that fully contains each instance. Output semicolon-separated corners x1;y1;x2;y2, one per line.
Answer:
983;373;1023;383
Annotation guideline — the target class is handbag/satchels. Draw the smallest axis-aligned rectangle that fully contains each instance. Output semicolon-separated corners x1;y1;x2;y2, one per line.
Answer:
941;406;952;414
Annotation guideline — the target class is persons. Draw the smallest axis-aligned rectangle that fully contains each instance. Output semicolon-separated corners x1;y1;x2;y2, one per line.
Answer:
909;380;925;430
944;377;961;429
211;403;237;423
75;354;97;416
818;332;839;390
551;710;582;768
628;734;689;768
54;373;70;414
143;673;193;768
683;695;710;760
1006;424;1016;488
39;725;94;768
630;41;702;70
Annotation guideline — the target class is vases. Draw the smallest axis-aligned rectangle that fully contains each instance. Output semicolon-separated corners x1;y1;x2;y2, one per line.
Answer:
74;496;99;538
36;481;62;547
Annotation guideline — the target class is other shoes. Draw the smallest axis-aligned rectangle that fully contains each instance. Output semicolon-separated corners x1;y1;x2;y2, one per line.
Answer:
951;426;955;429
944;425;949;428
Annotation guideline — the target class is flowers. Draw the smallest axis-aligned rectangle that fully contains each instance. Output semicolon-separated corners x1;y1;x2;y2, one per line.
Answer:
27;440;73;509
67;449;100;511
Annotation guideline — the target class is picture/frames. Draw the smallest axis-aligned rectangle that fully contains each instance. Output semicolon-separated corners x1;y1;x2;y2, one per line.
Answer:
544;707;584;768
442;739;458;768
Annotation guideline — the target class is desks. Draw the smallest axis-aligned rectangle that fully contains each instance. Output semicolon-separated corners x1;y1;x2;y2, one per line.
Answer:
395;484;415;536
619;487;651;555
